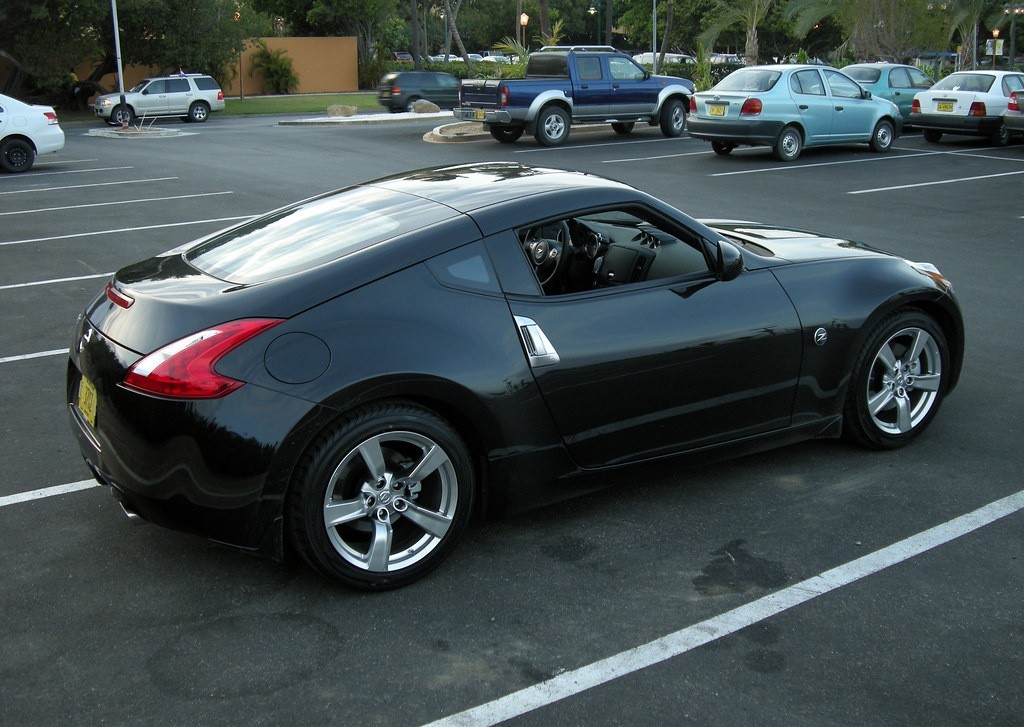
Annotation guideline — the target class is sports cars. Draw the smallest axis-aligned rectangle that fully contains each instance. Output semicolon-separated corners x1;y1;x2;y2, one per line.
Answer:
67;162;964;594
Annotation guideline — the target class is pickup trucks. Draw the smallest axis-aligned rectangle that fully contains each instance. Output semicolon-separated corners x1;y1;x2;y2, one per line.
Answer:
454;46;697;148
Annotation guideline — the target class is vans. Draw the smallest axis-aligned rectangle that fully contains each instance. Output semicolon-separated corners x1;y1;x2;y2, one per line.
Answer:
377;71;460;112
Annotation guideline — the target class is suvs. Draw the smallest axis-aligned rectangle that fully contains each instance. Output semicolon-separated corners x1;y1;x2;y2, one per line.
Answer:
94;74;225;125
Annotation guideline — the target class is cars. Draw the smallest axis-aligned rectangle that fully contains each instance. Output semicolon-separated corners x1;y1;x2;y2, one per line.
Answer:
610;52;831;65
0;93;66;173
1004;89;1024;144
388;50;529;64
909;70;1024;148
810;60;948;131
686;64;903;162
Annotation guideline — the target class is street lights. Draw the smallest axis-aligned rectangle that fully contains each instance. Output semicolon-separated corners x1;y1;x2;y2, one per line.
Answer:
439;13;448;50
1003;4;1024;70
991;28;1000;70
520;13;529;54
